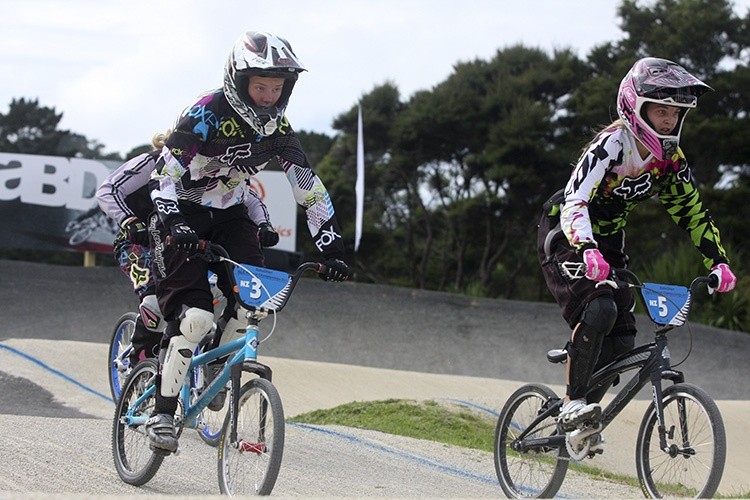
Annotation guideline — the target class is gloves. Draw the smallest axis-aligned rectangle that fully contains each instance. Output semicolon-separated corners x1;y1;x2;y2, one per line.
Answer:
705;264;737;295
168;220;204;253
254;222;280;250
124;218;150;246
583;249;611;282
323;257;350;286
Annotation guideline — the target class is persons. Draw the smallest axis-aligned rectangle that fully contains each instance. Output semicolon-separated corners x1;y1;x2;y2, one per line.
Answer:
75;205;100;223
537;56;739;456
148;28;350;458
94;127;281;375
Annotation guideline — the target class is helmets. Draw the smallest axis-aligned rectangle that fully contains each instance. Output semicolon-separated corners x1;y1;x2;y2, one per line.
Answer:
616;56;715;162
220;29;310;140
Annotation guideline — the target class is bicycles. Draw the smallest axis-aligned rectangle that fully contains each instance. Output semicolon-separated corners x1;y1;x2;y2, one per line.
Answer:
493;267;726;500
65;209;117;245
108;229;326;496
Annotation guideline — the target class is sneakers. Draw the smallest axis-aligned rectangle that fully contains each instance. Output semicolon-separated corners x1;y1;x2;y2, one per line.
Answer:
583;431;607;456
556;398;603;431
144;413;180;452
201;363;231;412
